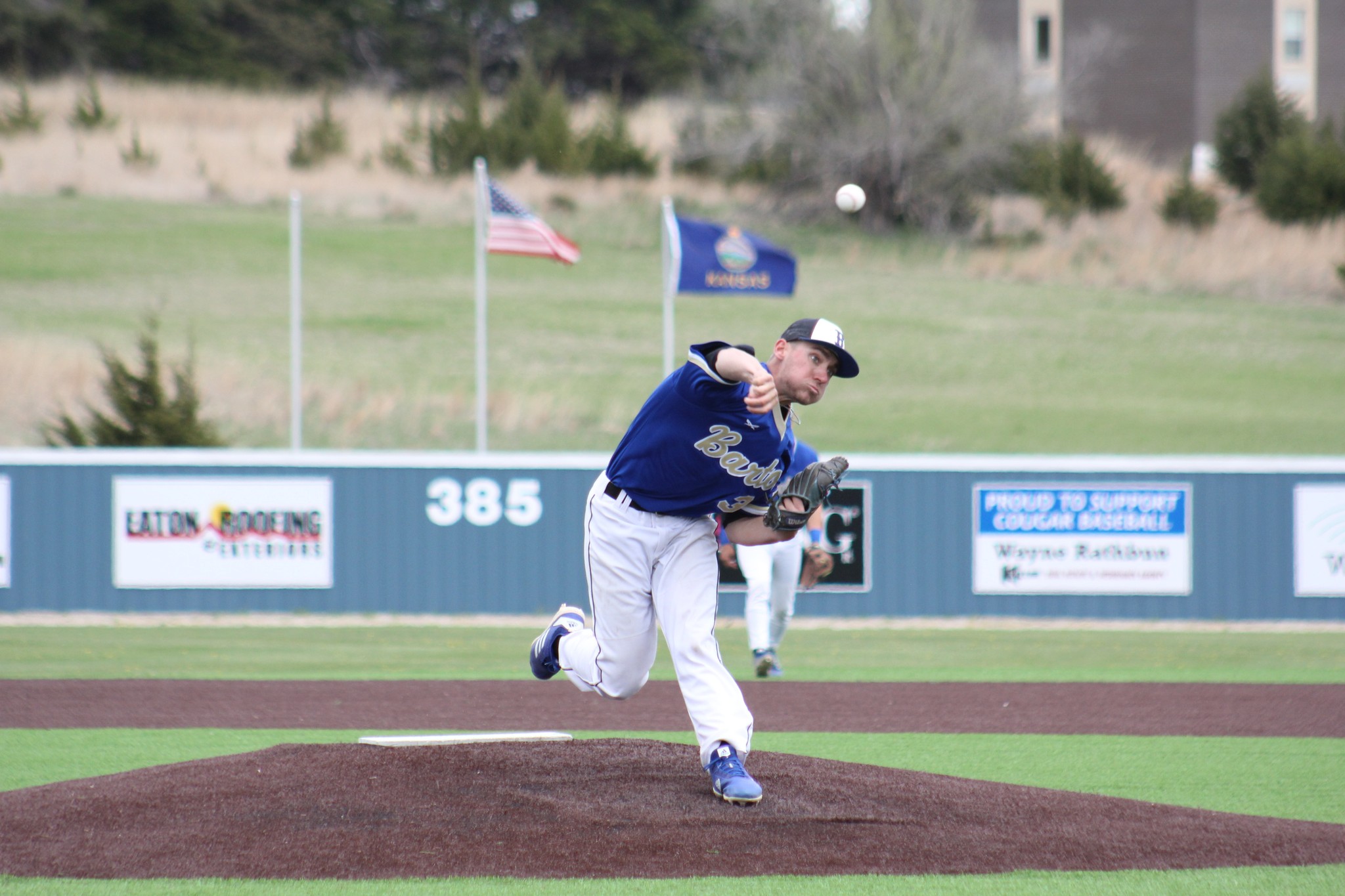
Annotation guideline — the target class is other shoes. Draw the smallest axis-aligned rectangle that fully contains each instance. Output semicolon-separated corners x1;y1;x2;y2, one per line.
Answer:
753;649;783;678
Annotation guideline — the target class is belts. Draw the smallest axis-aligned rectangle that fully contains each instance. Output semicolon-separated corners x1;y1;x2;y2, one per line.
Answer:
604;482;659;515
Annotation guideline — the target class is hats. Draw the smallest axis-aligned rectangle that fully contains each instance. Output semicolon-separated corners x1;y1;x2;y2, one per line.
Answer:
779;318;860;378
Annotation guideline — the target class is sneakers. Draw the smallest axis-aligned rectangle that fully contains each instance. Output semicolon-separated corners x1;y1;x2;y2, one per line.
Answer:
529;604;586;680
703;746;763;805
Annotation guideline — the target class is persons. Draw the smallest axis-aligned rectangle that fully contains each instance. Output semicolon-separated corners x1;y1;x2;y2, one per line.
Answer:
716;438;824;677
529;317;860;805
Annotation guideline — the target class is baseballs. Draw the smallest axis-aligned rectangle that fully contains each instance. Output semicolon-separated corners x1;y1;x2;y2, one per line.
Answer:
834;183;867;214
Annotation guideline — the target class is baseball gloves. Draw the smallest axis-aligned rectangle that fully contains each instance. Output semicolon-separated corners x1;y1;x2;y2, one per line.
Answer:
762;455;849;531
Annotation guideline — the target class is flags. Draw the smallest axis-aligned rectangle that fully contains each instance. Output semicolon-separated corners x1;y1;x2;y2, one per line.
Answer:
479;181;580;265
666;211;796;300
